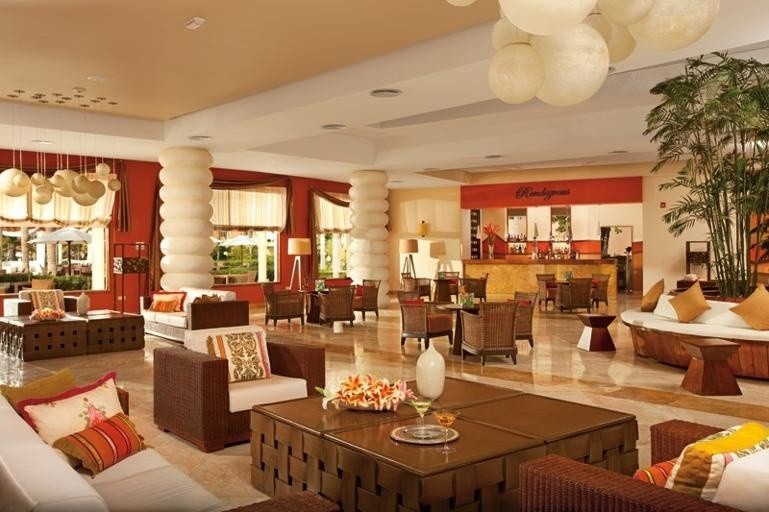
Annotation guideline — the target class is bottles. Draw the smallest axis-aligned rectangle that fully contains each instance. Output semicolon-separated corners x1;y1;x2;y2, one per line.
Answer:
510;233;526;254
531;237;580;259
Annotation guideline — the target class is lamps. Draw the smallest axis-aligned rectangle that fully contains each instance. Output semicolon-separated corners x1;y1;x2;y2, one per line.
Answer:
285;237;312;291
0;86;121;206
400;220;446;278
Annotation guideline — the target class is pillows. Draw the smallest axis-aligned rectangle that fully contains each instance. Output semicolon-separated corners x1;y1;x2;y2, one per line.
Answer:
0;371;145;479
151;291;186;311
634;421;768;512
640;277;769;329
207;331;272;382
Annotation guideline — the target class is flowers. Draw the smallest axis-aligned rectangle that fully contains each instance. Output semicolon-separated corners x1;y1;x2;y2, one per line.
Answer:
29;307;65;320
483;224;499;245
314;375;417;413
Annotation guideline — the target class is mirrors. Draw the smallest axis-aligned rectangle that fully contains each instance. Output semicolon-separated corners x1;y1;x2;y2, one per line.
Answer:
550;206;572;242
506;208;527;243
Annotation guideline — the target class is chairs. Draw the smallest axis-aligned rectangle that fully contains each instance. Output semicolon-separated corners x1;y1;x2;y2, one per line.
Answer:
153;324;326;452
214;269;260;284
517;420;769;512
4;289;78;315
40;264;91;275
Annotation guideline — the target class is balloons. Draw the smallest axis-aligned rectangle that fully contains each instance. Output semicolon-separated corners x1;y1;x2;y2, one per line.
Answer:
444;1;720;108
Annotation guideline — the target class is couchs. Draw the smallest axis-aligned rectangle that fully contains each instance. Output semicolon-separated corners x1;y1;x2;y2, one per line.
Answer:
140;287;249;344
0;368;342;512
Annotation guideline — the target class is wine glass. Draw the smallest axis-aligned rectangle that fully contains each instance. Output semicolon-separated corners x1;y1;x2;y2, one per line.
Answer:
432;408;462;455
411;397;433;438
0;329;24;361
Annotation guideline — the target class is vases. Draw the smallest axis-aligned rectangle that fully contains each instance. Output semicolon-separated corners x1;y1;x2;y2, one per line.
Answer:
76;291;91;316
489;245;495;261
415;338;446;398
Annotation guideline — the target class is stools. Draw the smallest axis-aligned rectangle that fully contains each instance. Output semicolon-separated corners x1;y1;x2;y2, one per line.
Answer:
679;338;744;396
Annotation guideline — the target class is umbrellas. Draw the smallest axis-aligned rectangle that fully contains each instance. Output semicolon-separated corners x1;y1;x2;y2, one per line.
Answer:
26;227;91;276
219;235;256;267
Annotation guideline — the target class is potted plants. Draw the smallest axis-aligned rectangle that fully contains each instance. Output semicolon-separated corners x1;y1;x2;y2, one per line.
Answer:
31;272;53;291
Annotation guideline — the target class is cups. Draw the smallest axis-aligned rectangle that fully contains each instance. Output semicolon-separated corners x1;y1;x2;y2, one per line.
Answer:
564;271;574;281
437;271;445;280
458;293;475;309
315;279;326;292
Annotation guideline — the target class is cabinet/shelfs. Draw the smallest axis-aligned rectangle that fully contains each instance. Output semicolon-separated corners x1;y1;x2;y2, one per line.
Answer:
113;243;150;311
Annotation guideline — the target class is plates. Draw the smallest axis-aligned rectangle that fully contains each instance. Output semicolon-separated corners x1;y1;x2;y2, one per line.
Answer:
389;423;460;446
